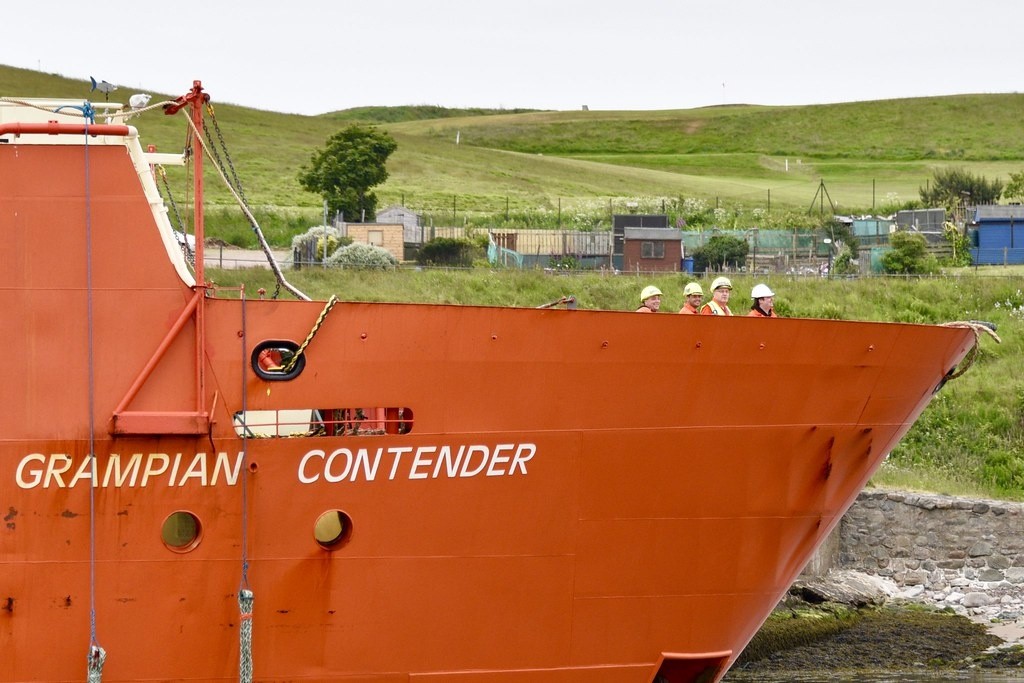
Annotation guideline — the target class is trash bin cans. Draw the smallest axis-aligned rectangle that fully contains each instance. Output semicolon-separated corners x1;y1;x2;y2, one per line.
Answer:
681;258;694;275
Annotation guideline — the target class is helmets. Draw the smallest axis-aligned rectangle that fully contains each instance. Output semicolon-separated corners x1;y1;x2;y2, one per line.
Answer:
683;283;703;296
710;277;732;293
640;286;662;302
751;284;774;298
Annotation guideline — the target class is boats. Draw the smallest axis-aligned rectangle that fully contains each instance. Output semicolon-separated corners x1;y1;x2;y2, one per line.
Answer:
0;73;1004;683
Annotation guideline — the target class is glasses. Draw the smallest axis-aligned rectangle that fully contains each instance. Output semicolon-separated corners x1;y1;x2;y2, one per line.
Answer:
716;290;729;293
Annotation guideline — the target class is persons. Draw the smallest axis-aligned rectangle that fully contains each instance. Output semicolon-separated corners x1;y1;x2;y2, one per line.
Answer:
635;285;664;313
746;283;778;317
700;277;734;316
678;282;703;314
